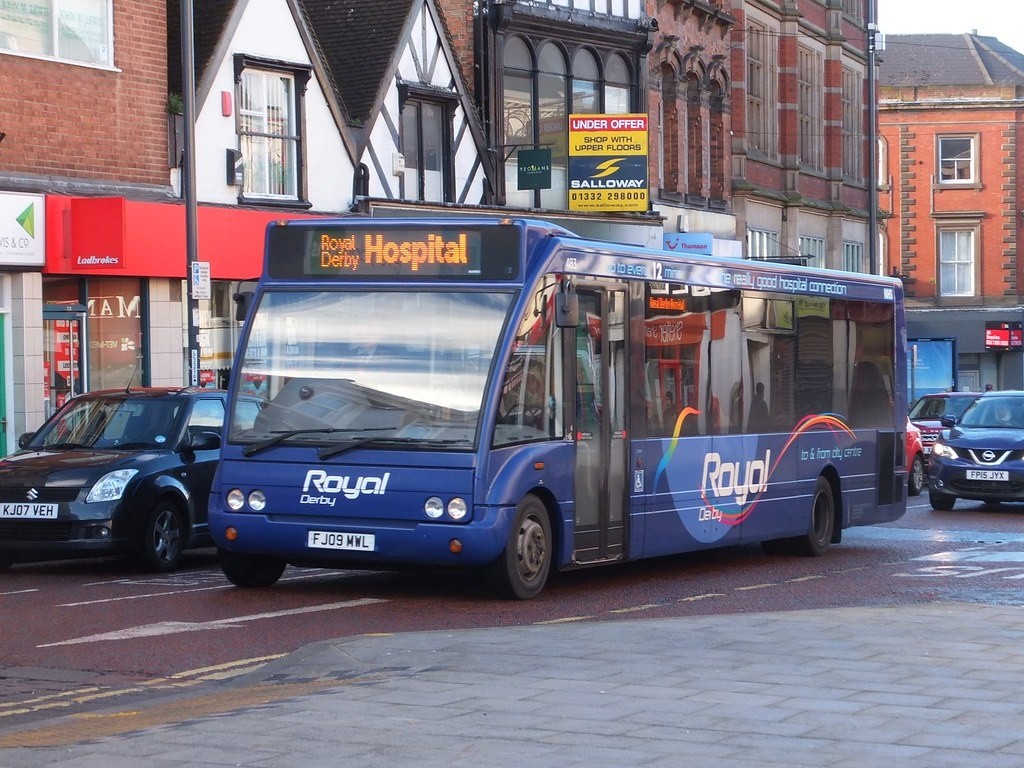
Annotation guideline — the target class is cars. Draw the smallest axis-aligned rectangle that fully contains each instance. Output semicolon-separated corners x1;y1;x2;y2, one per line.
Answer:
927;391;1024;510
0;387;269;572
907;392;983;474
907;416;926;494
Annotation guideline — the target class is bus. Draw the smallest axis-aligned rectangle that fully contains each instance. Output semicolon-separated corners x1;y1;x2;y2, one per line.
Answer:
208;218;907;600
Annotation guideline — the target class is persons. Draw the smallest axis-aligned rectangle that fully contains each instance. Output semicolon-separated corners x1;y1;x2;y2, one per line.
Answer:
995;401;1011;421
499;338;554;417
985;384;993;391
662;392;678;434
936;399;945;416
751;382;767;426
